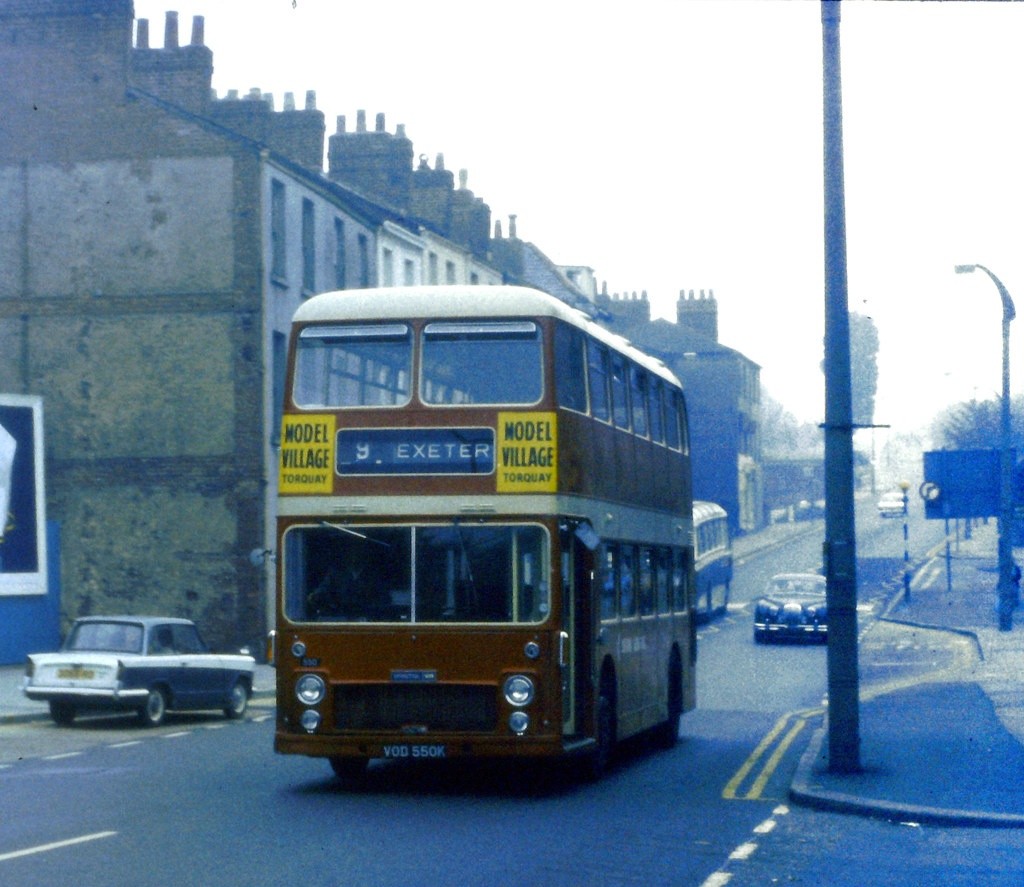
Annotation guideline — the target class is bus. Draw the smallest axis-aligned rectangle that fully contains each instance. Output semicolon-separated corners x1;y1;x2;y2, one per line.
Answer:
690;499;734;623
250;284;698;784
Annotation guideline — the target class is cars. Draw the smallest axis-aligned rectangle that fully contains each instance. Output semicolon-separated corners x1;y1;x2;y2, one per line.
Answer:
749;571;827;642
17;615;259;726
877;491;905;519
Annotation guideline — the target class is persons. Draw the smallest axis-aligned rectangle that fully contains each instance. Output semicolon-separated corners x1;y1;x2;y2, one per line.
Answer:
306;540;387;620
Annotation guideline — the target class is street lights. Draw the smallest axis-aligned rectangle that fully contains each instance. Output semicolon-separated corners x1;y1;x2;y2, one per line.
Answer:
900;479;912;598
954;263;1015;632
